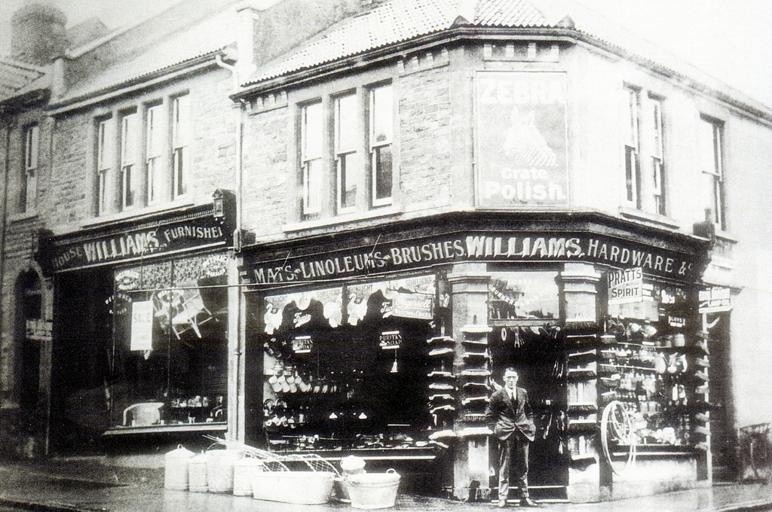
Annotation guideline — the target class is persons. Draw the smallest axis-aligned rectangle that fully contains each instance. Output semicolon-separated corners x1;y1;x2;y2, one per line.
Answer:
486;367;538;508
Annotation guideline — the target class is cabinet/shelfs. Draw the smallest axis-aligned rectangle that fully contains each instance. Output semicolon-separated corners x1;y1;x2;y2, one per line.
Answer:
269;375;307;393
262;404;311;430
614;390;639;413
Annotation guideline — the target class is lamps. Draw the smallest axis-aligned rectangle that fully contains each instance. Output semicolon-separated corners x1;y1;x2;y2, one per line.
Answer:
166;278;218;340
122;399;164;429
149;283;177;335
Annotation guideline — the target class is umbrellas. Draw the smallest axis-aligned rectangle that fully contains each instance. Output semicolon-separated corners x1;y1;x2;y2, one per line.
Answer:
497;499;507;507
520;497;537;507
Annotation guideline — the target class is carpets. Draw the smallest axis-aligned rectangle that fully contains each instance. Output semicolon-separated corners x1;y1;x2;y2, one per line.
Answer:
510;391;516;409
